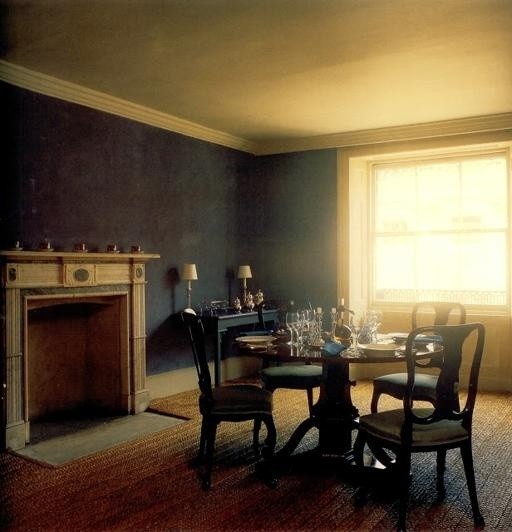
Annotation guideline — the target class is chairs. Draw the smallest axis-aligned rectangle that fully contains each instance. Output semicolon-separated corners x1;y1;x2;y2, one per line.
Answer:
370;302;466;415
353;322;486;532
257;298;324;415
180;307;277;492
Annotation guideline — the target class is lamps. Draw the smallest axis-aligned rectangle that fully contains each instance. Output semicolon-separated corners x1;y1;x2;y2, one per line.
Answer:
181;263;199;309
236;265;252;305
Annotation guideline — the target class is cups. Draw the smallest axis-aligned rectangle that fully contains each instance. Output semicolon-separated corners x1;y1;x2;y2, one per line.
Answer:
6;239;144;253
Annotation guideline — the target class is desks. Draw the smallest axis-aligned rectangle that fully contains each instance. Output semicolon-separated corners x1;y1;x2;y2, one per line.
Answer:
198;309;278;388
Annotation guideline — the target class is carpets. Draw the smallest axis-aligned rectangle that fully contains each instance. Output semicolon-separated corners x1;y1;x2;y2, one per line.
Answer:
0;377;512;532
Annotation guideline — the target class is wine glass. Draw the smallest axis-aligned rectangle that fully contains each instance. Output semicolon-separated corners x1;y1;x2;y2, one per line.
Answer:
283;308;381;356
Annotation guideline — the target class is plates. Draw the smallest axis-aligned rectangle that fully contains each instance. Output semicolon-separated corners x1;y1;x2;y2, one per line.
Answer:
236;335;277;347
391;331;436;345
356;344;404;357
